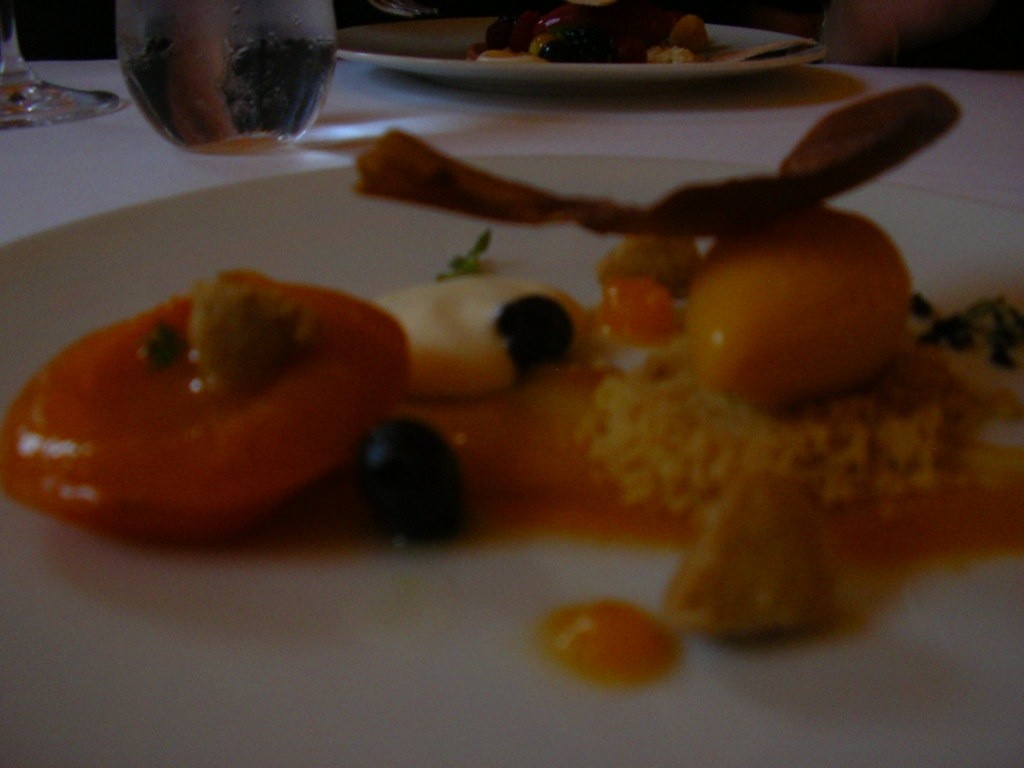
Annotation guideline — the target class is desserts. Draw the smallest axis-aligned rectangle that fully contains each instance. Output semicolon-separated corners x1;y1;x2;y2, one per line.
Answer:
1;81;973;585
464;0;714;67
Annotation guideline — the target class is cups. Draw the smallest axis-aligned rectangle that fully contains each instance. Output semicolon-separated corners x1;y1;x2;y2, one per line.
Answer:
114;0;337;156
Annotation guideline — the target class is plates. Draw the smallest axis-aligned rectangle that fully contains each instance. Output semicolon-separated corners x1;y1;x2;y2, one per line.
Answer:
335;15;826;91
0;155;1024;768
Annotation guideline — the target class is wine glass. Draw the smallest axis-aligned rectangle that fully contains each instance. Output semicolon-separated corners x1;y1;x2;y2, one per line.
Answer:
0;0;123;132
